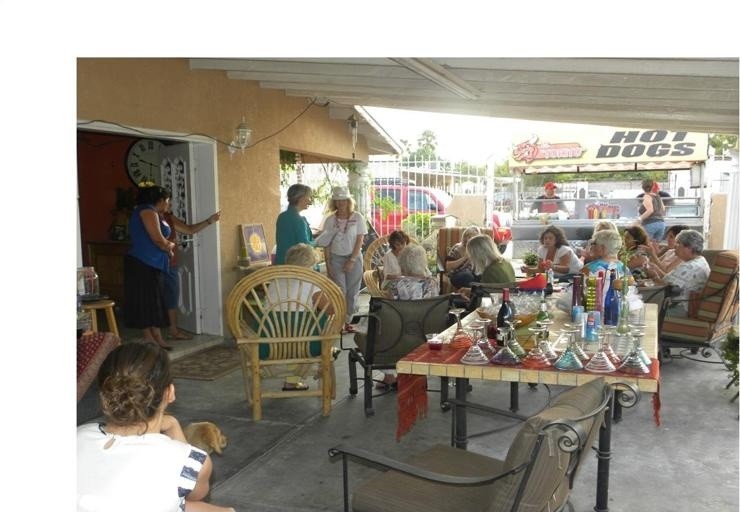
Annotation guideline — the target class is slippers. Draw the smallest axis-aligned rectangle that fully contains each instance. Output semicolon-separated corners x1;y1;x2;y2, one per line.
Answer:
281;382;310;392
376;380;397;390
345;323;354;331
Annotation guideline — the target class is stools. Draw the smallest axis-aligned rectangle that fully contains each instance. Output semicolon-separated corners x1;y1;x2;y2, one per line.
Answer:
81;300;122;349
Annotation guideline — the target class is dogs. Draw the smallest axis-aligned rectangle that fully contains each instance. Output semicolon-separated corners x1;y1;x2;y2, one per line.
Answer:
182;421;227;458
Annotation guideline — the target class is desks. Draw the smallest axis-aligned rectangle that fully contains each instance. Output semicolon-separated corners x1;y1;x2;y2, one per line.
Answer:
85;239;133;245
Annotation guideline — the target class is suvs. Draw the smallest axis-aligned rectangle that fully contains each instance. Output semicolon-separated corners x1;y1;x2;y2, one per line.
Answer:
370;178;512;258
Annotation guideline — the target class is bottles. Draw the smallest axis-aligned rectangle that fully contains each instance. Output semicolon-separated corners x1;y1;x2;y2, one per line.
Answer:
77;266;100;302
537;299;550;326
589;207;620;218
570;266;624;325
497;288;515;327
547;269;555;286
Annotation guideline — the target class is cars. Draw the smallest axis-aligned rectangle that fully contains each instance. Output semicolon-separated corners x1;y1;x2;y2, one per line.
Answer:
522;195;535;207
585;190;604;201
493;191;511;212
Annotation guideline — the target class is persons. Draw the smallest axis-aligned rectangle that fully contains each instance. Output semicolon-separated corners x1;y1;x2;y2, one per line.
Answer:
77;341;237;512
638;178;673;242
444;226;515;295
530;182;567;213
520;221;711;361
121;186;175;350
162;191;221;340
377;230;439;390
265;184;368;390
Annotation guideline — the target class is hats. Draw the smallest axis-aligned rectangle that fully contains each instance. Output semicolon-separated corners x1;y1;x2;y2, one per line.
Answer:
329;186;353;201
544;182;558;190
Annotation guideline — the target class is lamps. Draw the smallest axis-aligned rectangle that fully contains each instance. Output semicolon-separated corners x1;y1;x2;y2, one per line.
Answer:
227;114;252;156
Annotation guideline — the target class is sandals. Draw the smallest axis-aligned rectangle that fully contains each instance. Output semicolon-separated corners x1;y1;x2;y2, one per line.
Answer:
167;330;195;340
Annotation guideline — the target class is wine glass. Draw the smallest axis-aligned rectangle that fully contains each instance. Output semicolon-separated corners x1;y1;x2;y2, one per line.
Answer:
448;308;653;375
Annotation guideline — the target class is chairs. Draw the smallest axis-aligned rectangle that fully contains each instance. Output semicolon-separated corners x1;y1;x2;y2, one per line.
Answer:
328;378;641;512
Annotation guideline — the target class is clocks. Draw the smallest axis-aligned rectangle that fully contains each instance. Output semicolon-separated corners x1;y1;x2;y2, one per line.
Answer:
125;139;167;188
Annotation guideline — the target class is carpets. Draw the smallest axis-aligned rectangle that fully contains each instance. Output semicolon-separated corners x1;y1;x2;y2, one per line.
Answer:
169;342;253;381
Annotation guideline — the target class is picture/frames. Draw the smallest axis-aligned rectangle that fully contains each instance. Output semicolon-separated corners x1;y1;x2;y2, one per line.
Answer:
239;222;269;263
112;224;127;240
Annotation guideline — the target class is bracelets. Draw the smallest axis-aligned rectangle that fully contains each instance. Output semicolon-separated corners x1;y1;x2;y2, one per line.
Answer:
207;219;211;225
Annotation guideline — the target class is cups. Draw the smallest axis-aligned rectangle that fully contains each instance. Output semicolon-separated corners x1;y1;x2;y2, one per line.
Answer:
237;256;251;269
425;333;444;352
